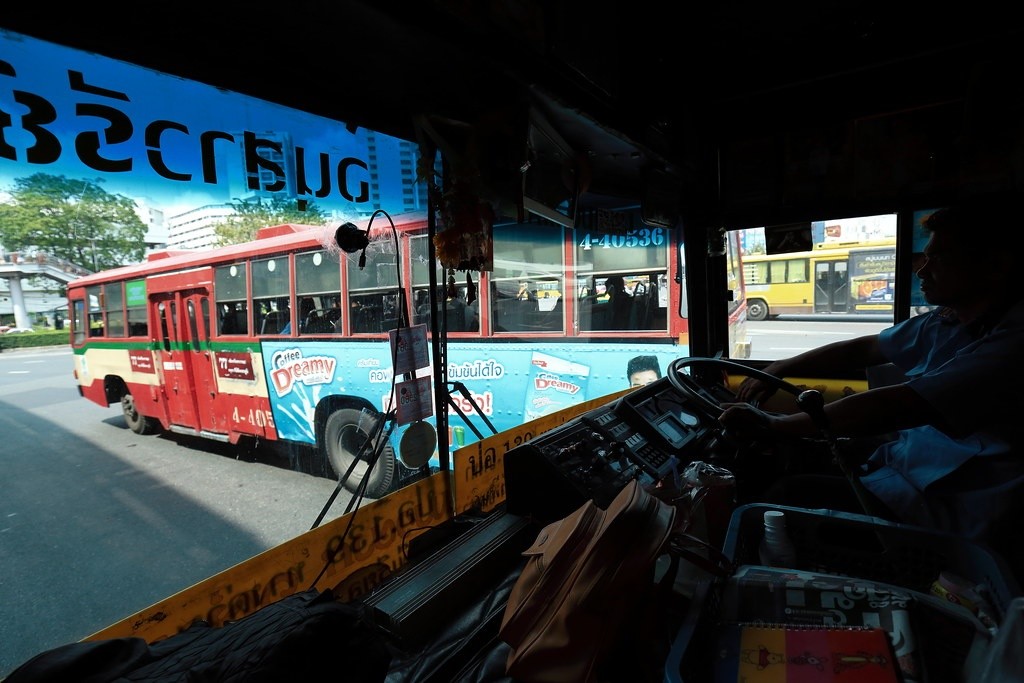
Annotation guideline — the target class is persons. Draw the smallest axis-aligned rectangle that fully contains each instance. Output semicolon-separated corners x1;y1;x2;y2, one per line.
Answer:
0;248;91;277
717;207;1024;539
54;311;64;330
626;355;662;388
224;285;465;335
541;279;563;332
603;276;633;330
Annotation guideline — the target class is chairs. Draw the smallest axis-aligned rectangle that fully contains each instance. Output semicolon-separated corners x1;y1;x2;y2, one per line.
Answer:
218;279;655;334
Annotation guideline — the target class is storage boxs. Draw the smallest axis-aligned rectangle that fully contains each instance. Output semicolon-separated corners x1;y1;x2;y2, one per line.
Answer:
666;502;1014;682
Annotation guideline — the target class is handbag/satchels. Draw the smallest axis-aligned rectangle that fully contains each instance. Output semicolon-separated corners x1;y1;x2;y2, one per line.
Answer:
673;460;736;611
409;570;523;683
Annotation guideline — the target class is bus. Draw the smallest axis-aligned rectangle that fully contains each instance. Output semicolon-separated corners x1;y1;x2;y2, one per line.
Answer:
54;206;753;498
520;274;652;301
742;239;896;322
437;259;592;337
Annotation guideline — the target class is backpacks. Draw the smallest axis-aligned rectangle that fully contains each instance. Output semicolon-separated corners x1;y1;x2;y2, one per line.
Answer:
2;587;417;683
497;478;677;683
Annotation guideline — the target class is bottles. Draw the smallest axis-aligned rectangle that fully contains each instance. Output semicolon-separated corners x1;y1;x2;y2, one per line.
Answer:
765;511;789;567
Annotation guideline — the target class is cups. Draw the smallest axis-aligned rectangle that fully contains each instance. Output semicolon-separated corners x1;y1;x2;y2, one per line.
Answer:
449;425;453;446
454;426;465;447
